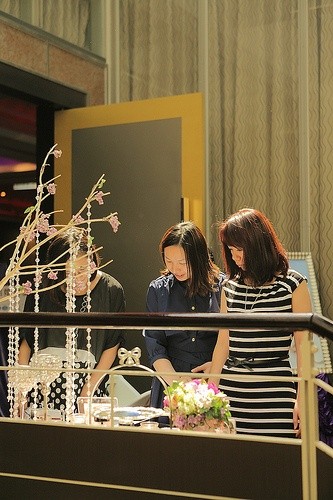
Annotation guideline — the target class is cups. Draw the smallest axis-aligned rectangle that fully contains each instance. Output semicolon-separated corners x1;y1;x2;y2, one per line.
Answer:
140;422;159;429
72;412;87;425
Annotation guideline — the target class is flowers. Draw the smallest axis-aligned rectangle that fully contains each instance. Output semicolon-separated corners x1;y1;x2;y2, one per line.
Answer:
0;142;122;302
162;377;234;434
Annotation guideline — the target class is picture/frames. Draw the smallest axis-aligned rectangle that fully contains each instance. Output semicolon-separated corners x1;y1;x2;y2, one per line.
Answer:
286;251;332;375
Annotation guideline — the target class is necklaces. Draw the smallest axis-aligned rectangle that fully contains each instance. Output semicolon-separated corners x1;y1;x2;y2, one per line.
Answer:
245;284;264;312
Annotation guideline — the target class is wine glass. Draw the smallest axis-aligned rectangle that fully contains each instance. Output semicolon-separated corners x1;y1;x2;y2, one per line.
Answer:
8;364;35;419
28;353;63;419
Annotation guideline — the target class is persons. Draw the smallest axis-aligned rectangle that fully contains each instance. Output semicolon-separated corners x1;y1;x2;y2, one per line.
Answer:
13;237;126;414
208;209;313;440
142;221;226;424
